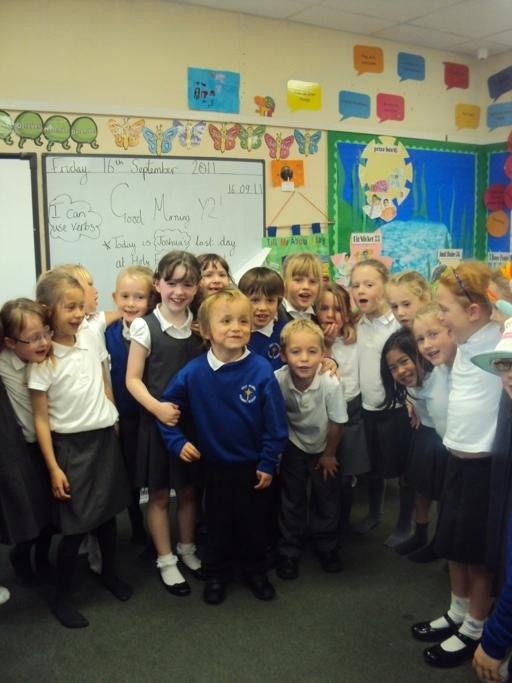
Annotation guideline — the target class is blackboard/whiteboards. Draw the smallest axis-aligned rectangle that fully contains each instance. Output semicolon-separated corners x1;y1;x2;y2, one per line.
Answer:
40;152;265;309
0;152;41;306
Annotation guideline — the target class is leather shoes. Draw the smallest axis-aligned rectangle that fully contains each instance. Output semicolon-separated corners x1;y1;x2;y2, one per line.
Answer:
424;633;478;667
319;551;343;573
204;578;224;604
246;578;274;600
178;550;209;580
411;612;458;640
278;557;299;580
157;559;192;598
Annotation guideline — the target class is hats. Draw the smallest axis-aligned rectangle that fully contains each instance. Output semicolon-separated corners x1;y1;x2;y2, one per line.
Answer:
471;316;512;377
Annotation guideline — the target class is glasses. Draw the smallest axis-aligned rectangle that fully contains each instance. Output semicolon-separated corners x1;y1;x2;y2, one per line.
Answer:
8;329;56;348
493;360;511;371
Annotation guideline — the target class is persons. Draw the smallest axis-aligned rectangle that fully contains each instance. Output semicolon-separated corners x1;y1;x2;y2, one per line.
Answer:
274;250;356;344
27;274;130;629
270;322;349;582
383;267;434;327
239;266;338;372
347;259;417;552
409;304;455;438
124;251;210;595
408;262;499;670
485;266;512;332
463;319;512;683
106;266;159;552
384;330;446;572
193;253;229;304
156;289;288;604
48;262;120;573
299;283;366;547
0;298;57;584
